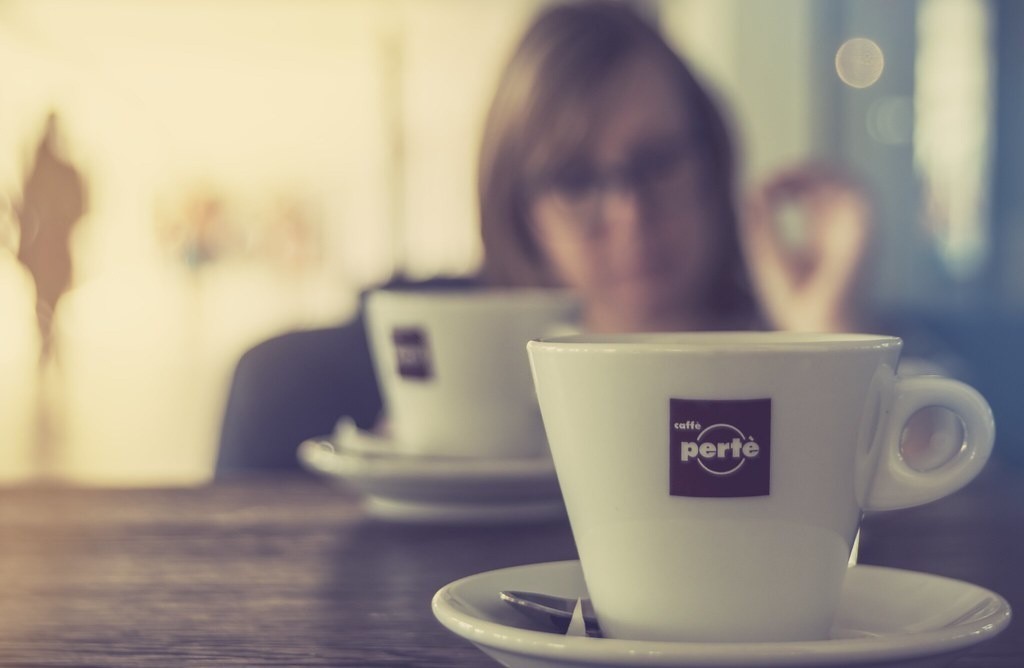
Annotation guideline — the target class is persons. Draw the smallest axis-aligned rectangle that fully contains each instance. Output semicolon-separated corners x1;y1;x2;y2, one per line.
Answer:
212;0;956;489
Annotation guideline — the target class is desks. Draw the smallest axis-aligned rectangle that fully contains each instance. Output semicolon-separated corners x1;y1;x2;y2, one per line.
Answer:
0;479;1024;668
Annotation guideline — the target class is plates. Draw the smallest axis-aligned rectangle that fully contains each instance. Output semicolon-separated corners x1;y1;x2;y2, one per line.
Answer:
298;435;571;524
432;560;1024;668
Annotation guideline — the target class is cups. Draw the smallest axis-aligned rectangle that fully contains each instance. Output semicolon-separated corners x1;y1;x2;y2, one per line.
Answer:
526;334;995;639
364;289;581;459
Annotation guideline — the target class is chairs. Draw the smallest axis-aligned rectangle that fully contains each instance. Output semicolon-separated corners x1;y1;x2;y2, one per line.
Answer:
206;325;383;481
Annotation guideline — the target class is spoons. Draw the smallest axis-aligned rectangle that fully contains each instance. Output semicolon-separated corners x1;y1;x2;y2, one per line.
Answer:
500;590;600;624
